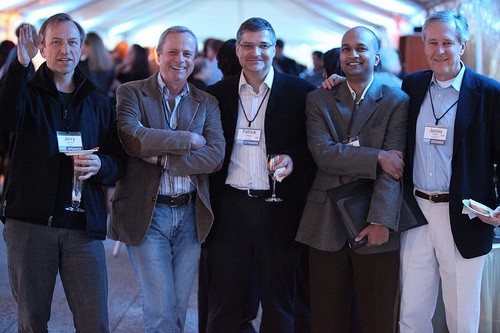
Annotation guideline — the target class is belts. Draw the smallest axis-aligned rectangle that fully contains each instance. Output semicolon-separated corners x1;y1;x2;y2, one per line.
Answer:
415;190;448;203
157;192;195;205
225;184;272;198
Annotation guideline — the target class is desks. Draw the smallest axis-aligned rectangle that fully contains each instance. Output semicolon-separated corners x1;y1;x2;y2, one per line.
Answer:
433;243;500;333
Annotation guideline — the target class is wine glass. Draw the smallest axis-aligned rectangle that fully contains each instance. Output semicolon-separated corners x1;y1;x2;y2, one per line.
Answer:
265;153;282;202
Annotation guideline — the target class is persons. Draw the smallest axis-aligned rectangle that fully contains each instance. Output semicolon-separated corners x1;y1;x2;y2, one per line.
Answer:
0;13;128;333
321;9;500;333
0;23;35;80
275;39;346;86
204;17;317;333
373;25;401;74
187;39;243;90
114;26;226;333
295;26;411;333
79;33;159;95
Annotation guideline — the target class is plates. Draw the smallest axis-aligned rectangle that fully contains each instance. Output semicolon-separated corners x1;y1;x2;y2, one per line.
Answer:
59;149;98;155
462;199;498;219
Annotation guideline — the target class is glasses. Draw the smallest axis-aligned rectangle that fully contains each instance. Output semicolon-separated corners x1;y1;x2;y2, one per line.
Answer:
239;42;274;50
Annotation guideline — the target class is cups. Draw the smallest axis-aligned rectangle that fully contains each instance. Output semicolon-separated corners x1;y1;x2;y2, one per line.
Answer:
65;155;87;212
